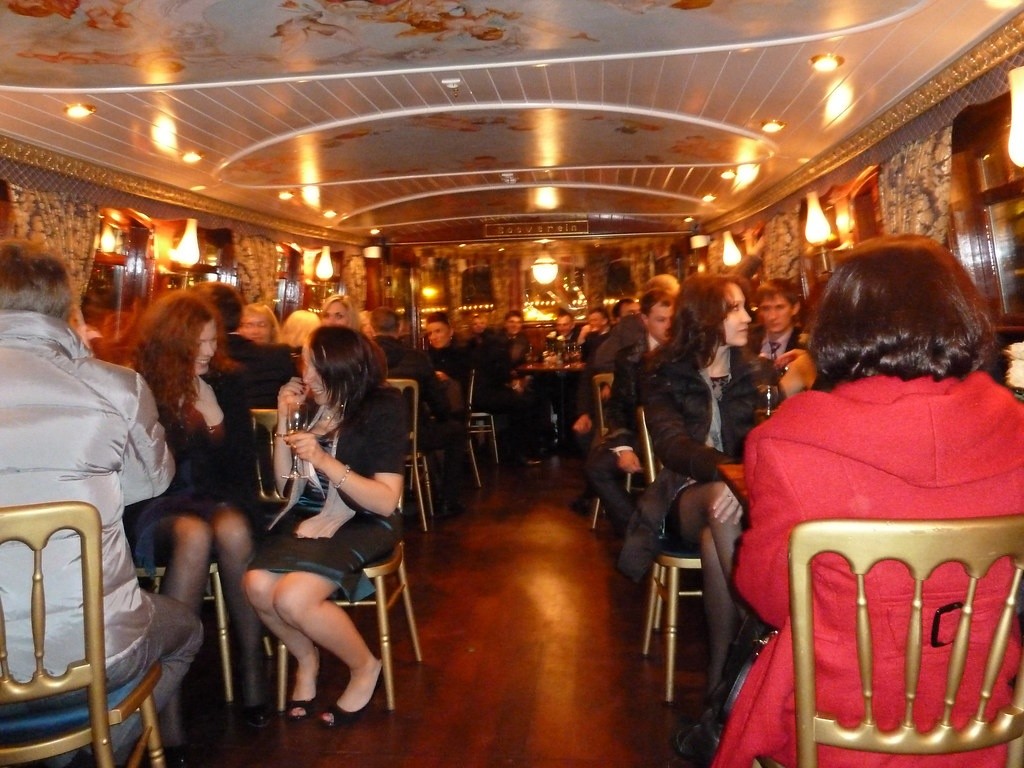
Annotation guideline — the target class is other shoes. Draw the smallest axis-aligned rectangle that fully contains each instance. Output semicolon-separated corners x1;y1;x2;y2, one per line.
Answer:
241;697;273;728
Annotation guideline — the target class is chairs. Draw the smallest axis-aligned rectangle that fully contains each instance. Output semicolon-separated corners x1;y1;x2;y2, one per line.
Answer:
588;371;637;534
633;403;706;709
244;403;300;504
470;410;499;466
130;553;236;716
0;499;169;768
751;513;1024;768
456;365;483;488
385;376;437;532
276;536;425;716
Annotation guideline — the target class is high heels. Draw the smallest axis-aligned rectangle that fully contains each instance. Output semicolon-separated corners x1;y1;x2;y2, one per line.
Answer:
322;664;385;727
289;650;325;722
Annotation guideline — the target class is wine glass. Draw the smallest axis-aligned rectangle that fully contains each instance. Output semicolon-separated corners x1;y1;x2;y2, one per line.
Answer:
527;340;582;368
281;402;309;479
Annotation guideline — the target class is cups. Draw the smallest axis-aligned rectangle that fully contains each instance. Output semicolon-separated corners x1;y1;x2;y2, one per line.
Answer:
757;383;779;418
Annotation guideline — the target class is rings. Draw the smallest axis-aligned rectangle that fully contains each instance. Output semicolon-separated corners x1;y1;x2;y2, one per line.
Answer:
728;495;733;503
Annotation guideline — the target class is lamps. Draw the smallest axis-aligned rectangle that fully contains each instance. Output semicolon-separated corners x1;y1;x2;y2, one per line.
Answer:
1007;64;1024;170
718;228;743;270
528;239;559;284
160;214;199;291
804;189;854;279
306;240;335;310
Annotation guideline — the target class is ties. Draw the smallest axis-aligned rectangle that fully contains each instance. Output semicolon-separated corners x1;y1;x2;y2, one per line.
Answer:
769;340;782;361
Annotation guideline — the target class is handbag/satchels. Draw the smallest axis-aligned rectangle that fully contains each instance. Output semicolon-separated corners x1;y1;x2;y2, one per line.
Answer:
675;618;778;759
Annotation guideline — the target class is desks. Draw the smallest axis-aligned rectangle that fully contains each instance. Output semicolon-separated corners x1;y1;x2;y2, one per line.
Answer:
518;360;588;454
712;462;756;522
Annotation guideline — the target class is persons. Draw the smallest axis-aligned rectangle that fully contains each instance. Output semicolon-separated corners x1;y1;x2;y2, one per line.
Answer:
115;238;1024;768
0;238;202;768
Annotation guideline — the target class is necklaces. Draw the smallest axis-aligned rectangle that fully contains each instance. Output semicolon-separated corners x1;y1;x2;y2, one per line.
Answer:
321;408;340;421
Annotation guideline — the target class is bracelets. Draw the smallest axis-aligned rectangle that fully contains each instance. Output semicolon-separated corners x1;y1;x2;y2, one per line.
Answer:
273;433;287;438
332;464;350;489
207;420;224;431
